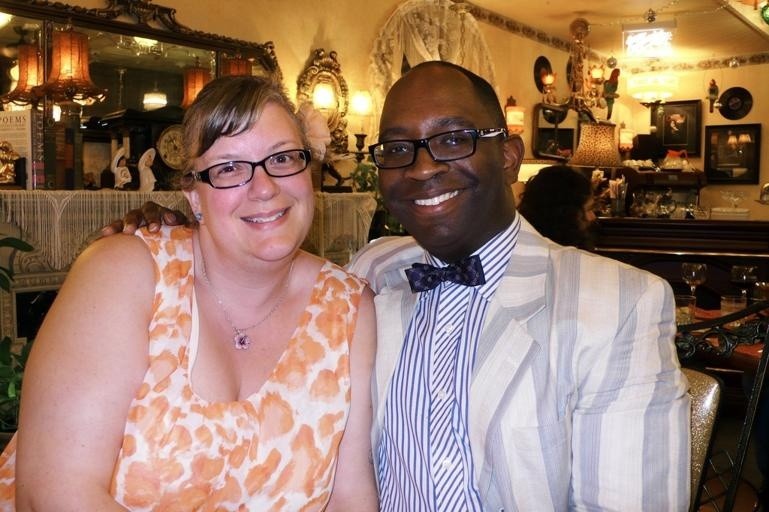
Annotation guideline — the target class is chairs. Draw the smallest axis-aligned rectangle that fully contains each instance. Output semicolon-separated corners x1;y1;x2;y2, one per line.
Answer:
676;365;726;511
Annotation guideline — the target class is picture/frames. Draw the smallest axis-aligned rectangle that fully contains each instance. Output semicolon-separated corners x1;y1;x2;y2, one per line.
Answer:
648;98;762;186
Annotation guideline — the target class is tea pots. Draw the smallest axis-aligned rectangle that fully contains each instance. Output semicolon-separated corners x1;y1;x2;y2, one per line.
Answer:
662;149;688;174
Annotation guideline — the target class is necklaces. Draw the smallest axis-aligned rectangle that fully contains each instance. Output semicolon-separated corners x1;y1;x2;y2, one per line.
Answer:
204;257;294;349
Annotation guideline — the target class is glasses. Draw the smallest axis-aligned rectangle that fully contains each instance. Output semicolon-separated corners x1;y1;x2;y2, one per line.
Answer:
184;149;312;189
370;127;508;169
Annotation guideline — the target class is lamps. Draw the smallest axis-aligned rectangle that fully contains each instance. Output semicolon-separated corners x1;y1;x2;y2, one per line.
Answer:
3;20;109;116
540;17;606;111
176;50;255;110
566;122;626;197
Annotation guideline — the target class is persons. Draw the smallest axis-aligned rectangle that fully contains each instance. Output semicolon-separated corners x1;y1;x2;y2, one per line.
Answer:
0;76;381;509
524;164;608;248
343;61;698;512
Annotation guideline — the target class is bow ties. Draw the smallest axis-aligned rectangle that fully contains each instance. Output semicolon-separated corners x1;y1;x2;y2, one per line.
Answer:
405;255;485;294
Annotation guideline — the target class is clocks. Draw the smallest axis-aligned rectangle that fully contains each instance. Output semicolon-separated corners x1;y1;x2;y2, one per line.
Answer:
155;123;192;172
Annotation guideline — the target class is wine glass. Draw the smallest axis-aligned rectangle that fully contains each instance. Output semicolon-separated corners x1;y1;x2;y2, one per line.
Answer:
730;264;757;297
681;262;707;295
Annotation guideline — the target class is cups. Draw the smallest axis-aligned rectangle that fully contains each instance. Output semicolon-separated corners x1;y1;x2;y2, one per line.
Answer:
602;177;753;222
674;295;696;325
720;295;747;330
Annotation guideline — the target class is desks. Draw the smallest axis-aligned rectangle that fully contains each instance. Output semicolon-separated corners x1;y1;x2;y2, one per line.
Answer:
669;301;767;383
